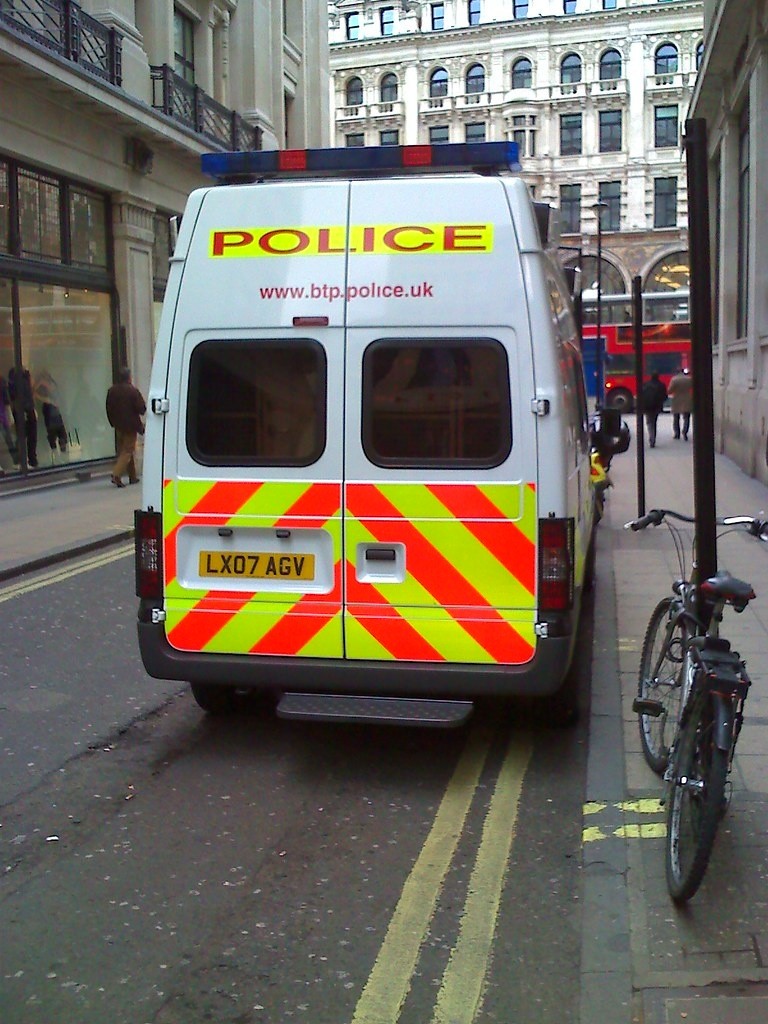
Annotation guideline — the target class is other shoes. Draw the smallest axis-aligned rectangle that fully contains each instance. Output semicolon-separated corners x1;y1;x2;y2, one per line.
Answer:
112;477;124;487
129;479;139;484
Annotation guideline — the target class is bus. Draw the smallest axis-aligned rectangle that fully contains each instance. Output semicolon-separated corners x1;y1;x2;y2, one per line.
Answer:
572;285;694;415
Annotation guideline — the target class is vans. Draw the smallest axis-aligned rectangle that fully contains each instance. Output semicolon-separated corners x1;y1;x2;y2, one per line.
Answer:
134;141;621;734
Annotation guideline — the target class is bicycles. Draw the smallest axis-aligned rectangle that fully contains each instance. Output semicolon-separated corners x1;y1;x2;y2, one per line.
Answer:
623;507;768;905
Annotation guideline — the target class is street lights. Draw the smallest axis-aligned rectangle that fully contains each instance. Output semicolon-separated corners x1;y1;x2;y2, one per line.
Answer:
589;191;609;407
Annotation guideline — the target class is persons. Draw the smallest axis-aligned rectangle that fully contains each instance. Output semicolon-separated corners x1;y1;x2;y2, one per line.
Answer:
642;370;668;447
0;374;20;474
622;309;651;322
8;364;42;473
32;368;71;464
105;367;147;488
667;367;693;441
